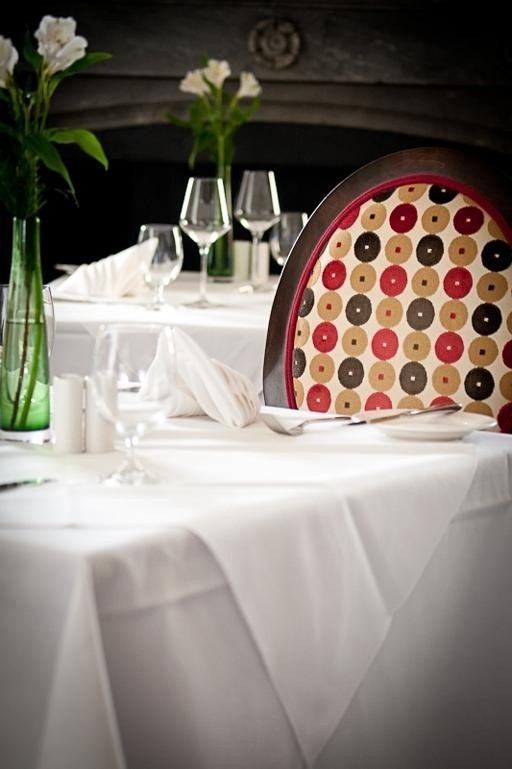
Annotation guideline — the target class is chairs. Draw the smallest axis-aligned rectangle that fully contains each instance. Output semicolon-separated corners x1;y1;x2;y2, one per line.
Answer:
263;144;510;435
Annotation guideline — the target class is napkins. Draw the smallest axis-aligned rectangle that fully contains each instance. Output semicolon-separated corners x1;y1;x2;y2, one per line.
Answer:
137;326;261;428
53;238;160;303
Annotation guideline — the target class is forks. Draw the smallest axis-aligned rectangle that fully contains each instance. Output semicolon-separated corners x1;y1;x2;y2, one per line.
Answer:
260;410;352;438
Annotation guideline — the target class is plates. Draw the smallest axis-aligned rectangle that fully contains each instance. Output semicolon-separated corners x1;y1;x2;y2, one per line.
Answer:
350;408;498;441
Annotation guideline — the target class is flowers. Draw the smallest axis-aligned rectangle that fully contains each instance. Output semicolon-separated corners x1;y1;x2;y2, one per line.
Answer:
164;57;263;275
1;13;114;429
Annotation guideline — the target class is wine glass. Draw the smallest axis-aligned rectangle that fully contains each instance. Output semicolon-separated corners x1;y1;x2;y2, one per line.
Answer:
92;324;180;496
269;213;308;265
137;224;183;312
179;177;232;309
233;171;281;296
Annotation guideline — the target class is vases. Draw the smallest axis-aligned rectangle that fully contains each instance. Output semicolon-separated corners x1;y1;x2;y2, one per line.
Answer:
2;216;51;440
207;164;237;284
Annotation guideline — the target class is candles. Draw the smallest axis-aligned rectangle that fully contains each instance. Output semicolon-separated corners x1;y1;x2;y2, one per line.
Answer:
51;375;86;454
86;375;121;453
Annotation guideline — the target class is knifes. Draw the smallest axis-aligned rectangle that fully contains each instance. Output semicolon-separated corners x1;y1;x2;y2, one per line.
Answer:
342;402;462;429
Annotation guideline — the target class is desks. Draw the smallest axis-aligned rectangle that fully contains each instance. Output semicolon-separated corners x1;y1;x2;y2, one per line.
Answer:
0;379;510;768
38;265;283;382
